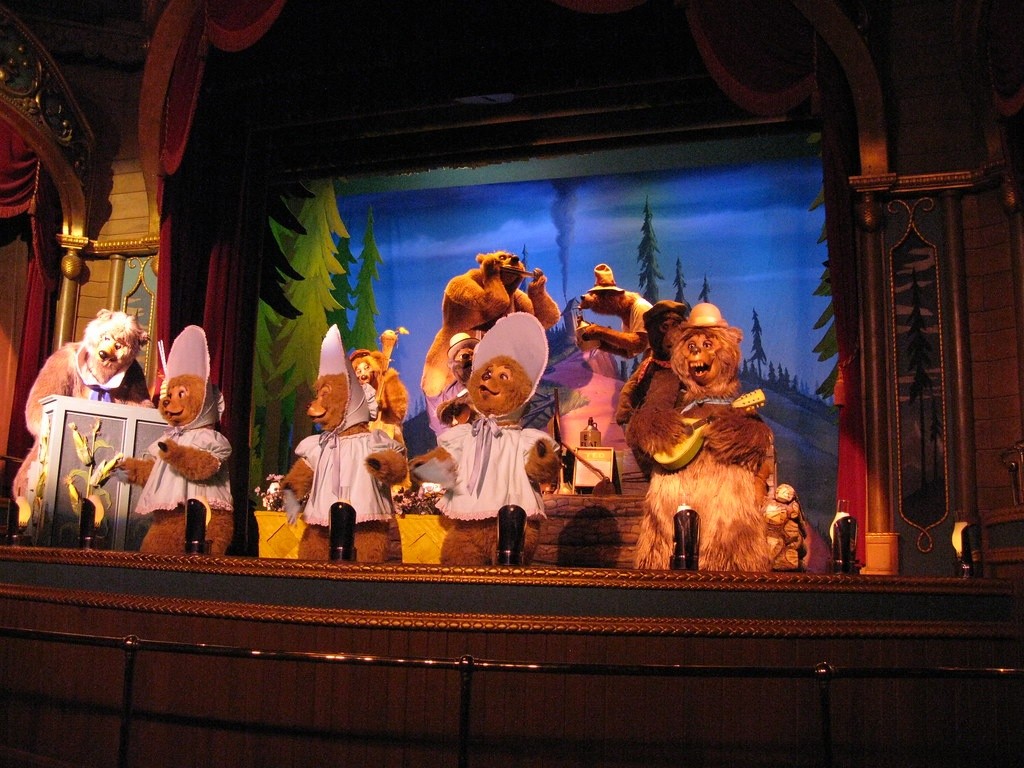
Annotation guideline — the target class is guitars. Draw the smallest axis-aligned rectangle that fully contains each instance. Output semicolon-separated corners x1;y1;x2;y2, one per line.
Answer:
651;388;768;471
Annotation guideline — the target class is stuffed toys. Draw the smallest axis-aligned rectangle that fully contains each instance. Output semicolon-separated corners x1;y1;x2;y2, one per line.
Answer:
13;309;153;499
350;251;687;495
409;312;561;566
116;325;232;556
275;323;407;564
634;302;771;571
